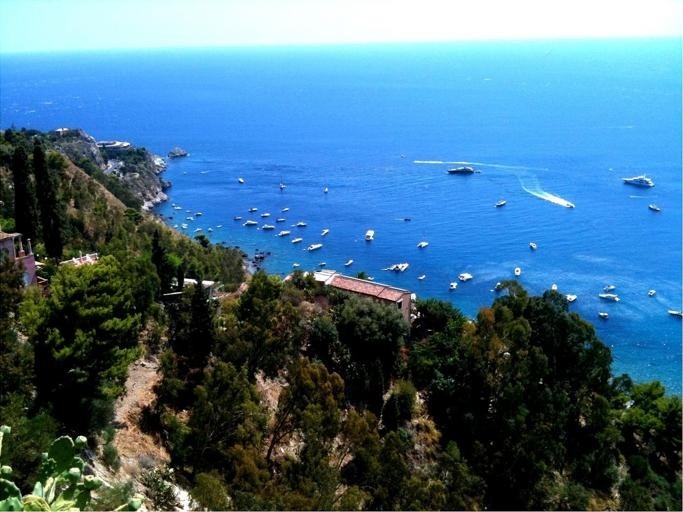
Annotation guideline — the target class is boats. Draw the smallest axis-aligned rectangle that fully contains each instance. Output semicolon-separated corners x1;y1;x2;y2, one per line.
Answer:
650;204;659;210
648;290;655;296
239;177;244;183
496;201;505;205
345;260;353;266
394;265;402;272
169;204;223;233
233;208;329;250
418;242;428;247
515;267;519;275
669;310;682;316
531;242;536;249
552;284;576;301
449;273;472;290
449;166;473;173
418;275;425;279
366;230;375;240
598;285;619;319
625;177;654;187
